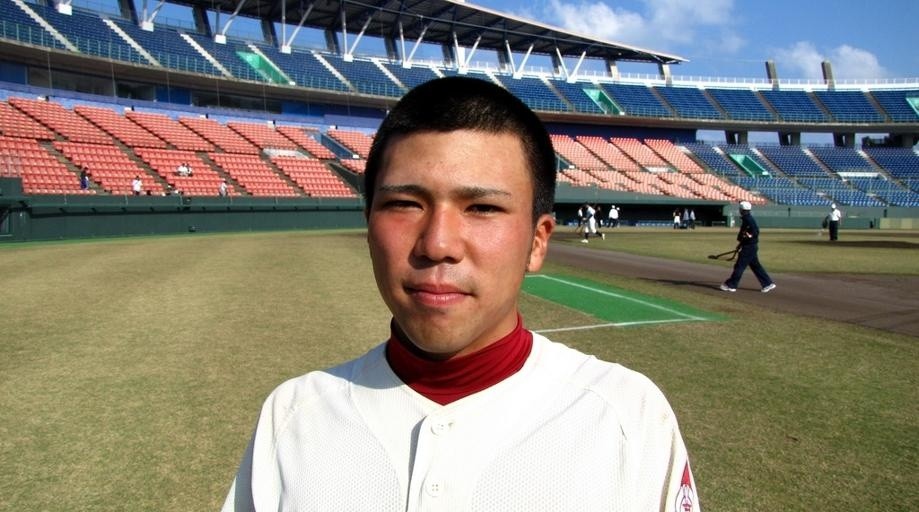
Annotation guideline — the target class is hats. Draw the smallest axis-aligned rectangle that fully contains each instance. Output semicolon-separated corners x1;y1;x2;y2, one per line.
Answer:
581;239;588;243
831;204;836;208
739;202;752;210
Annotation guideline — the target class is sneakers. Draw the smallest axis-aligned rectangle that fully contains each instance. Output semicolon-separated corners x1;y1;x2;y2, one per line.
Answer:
720;283;736;292
602;234;605;240
761;283;776;293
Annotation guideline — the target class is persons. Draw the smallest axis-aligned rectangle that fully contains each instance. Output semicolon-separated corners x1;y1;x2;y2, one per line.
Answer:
719;200;776;293
825;203;841;240
672;208;696;230
130;175;144;197
176;162;194;178
80;166;90;190
576;203;622;244
218;75;699;511
219;179;229;196
